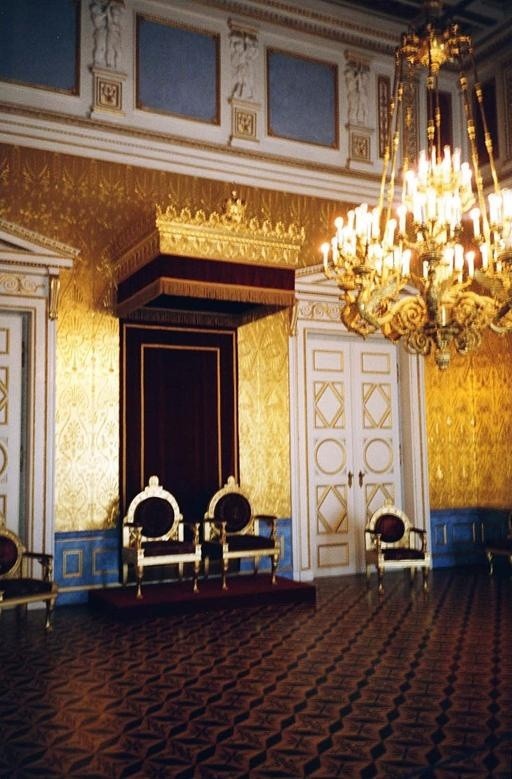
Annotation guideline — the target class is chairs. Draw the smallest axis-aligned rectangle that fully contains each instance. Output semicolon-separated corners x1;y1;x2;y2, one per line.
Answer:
117;474;204;600
361;496;431;599
481;510;512;577
199;474;282;593
1;510;59;635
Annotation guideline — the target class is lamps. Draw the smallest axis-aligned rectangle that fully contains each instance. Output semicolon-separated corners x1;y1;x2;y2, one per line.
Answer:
314;1;511;373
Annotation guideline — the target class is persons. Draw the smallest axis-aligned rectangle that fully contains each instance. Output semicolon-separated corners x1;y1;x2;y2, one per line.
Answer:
226;189;246;223
344;69;371;128
229;33;260;102
89;1;123;71
